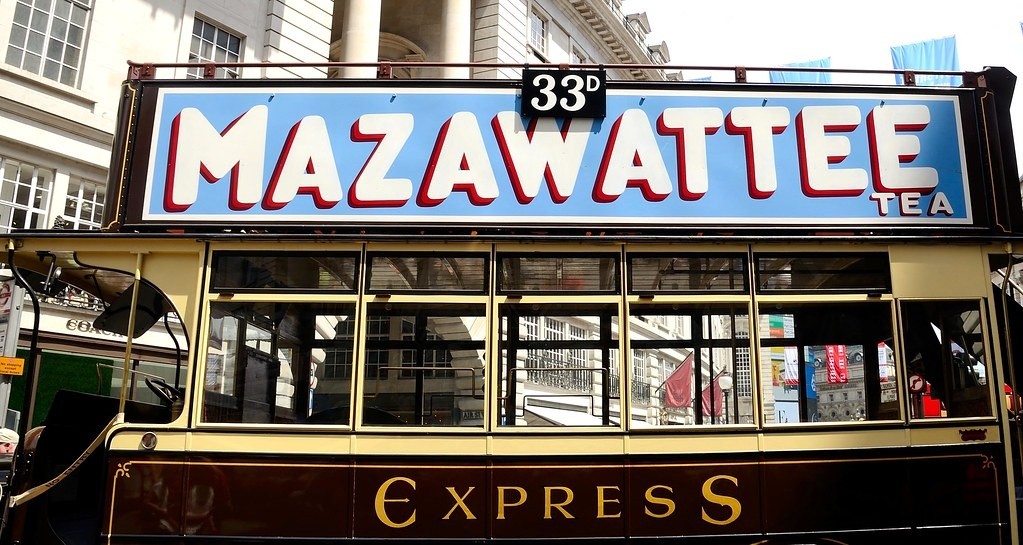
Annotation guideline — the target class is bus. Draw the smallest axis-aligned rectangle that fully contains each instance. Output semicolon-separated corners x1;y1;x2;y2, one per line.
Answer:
0;59;1023;545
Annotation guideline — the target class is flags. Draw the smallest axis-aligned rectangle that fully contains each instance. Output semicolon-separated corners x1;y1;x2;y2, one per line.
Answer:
663;352;694;409
878;343;888;384
700;369;726;417
825;345;849;385
784;346;799;386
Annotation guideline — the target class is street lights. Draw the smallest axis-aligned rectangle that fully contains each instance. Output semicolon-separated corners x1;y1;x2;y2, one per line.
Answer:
718;371;732;424
